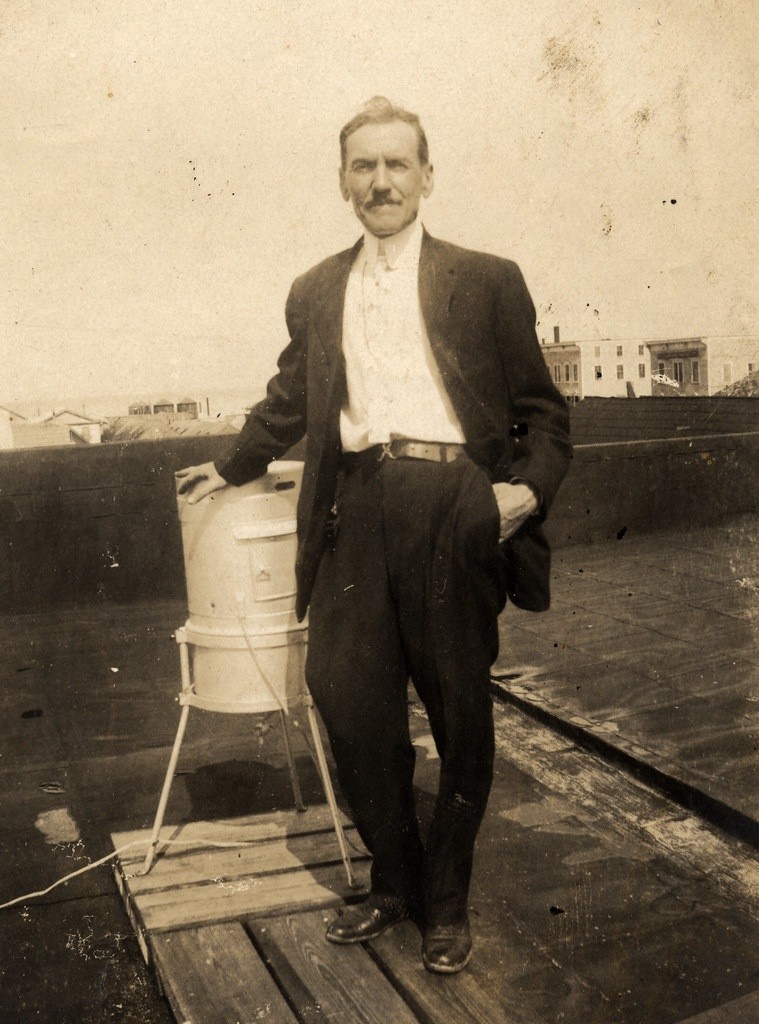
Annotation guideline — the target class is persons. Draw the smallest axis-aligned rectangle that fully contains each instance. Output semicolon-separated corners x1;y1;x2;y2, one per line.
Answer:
179;92;573;975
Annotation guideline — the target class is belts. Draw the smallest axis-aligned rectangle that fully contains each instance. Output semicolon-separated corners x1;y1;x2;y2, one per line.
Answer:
366;440;464;462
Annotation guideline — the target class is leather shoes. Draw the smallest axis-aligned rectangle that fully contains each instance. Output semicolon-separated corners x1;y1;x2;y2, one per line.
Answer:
326;890;417;944
423;925;473;973
